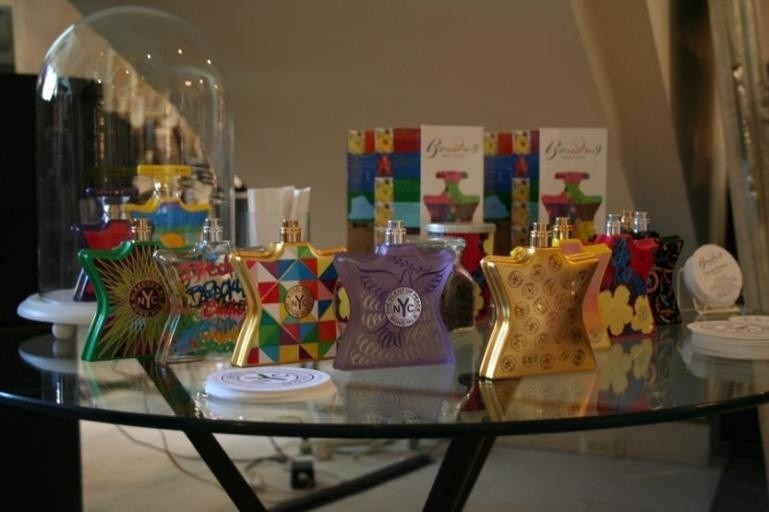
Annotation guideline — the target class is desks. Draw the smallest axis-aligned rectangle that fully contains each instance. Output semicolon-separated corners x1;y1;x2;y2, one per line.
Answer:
0;325;769;512
